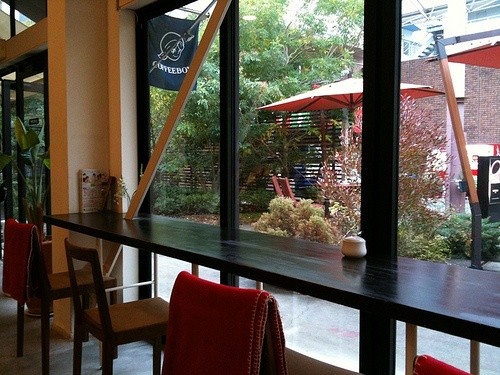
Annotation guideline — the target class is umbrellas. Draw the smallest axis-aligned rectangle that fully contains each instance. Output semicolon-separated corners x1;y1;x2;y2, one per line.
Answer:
254;76;446;131
423;38;500;69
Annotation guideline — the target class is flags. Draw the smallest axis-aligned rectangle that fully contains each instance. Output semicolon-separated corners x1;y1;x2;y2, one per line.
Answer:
148;15;199;91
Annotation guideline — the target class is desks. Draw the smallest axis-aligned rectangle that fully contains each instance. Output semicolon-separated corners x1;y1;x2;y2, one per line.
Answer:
42;209;500;348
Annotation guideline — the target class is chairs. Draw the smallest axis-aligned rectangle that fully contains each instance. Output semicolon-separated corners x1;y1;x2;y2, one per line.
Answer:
2;218;118;375
272;175;324;208
161;271;363;375
63;237;170;375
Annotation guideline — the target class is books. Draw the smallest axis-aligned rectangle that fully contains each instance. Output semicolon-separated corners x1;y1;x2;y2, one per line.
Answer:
79;167;110;215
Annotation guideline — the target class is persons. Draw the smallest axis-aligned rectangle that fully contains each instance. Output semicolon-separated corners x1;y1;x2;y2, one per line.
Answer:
316;162;326;187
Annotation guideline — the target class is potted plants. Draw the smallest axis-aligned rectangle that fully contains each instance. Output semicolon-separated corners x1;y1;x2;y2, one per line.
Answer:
0;115;53;314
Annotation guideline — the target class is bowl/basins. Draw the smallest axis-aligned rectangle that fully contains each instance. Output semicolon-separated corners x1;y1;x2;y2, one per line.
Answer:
342;233;368;257
